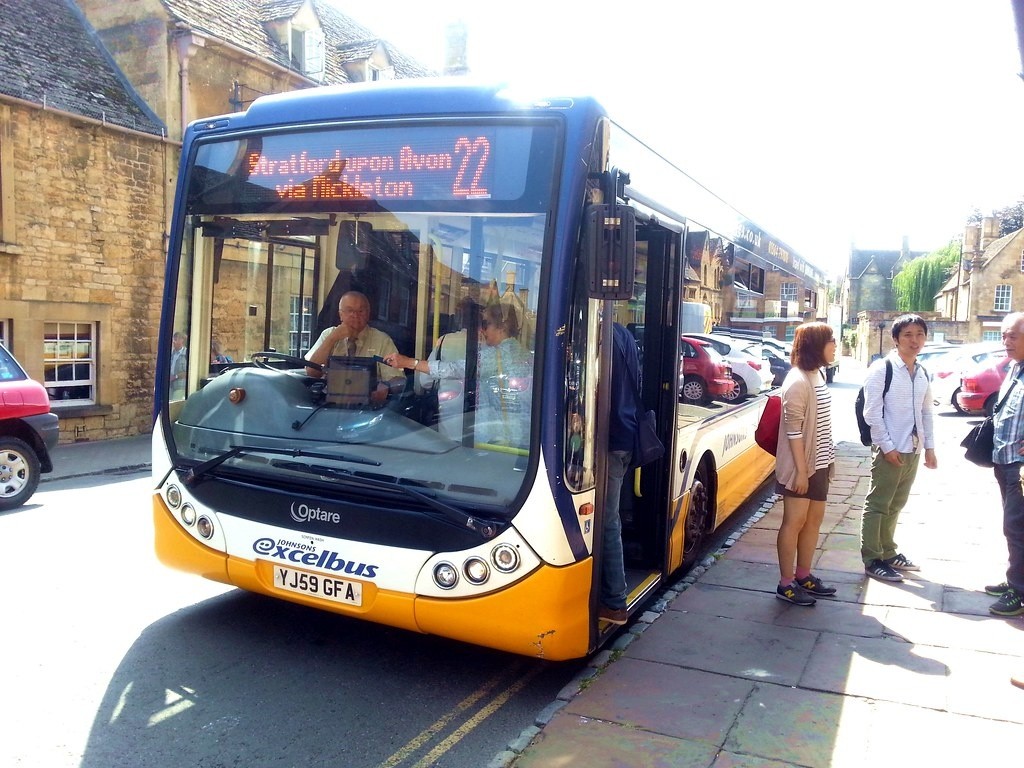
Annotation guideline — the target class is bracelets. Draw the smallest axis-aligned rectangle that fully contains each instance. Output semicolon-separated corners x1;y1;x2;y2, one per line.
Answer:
414;359;419;369
383;381;391;394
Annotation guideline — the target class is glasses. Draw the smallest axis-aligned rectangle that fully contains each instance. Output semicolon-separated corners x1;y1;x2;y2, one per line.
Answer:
339;306;367;316
481;320;491;331
825;338;837;346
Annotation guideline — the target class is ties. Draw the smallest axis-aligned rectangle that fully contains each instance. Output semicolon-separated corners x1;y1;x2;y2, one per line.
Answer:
348;336;357;357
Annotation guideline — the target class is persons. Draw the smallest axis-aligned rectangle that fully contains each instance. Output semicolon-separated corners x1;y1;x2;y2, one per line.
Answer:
985;312;1024;615
170;332;187;401
304;292;407;402
382;304;532;445
419;297;487;443
860;314;938;581
573;320;643;625
774;323;837;607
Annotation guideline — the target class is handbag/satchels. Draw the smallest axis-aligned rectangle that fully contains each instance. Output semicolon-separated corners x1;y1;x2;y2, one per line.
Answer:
400;394;434;426
630;410;665;469
962;416;994;468
755;370;824;457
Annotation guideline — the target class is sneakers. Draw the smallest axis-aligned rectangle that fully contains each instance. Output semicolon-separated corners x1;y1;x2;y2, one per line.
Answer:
991;588;1024;616
795;573;836;595
884;554;919;570
597;607;627;625
779;581;816;606
986;583;1011;596
868;561;903;581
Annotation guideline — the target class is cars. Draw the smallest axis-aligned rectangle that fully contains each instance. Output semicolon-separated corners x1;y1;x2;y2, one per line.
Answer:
681;326;795;405
0;342;60;510
892;340;1013;418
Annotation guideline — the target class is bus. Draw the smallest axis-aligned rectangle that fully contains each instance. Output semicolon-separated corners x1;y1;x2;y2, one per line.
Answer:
150;75;832;660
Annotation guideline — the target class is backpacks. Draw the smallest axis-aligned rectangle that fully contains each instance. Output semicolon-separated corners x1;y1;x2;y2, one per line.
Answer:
855;360;929;446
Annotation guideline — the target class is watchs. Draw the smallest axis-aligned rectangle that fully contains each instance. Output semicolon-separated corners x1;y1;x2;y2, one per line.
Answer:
175;374;179;380
